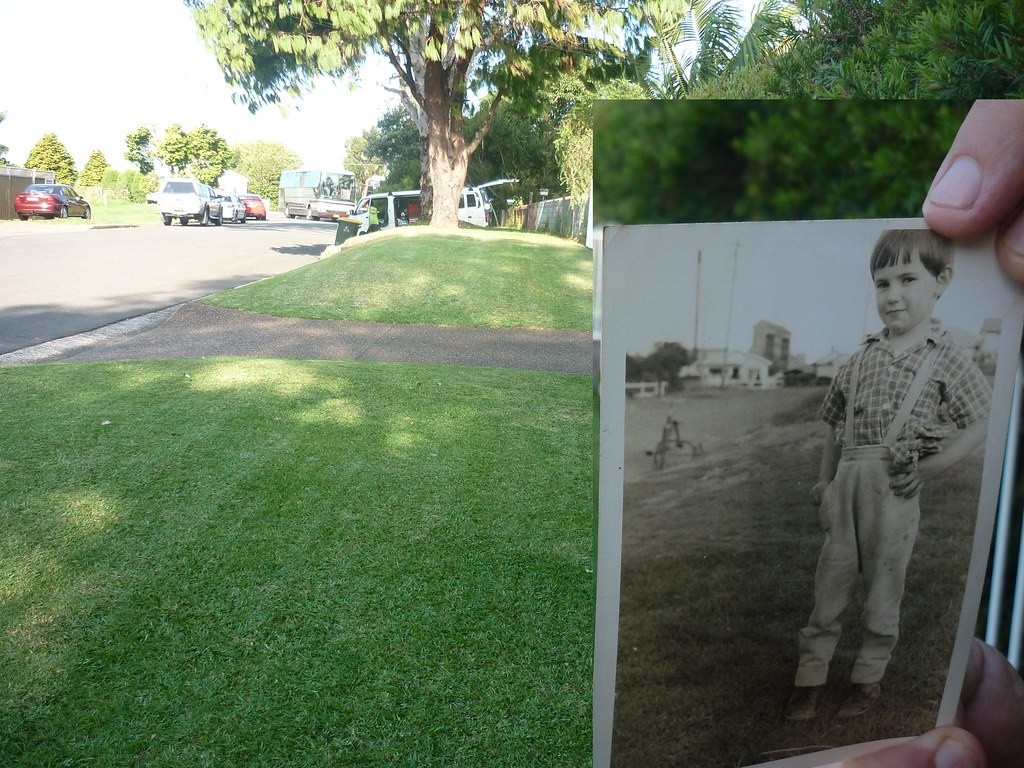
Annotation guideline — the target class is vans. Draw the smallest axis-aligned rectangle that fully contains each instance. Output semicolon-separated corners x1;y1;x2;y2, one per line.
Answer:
157;180;225;226
347;178;521;238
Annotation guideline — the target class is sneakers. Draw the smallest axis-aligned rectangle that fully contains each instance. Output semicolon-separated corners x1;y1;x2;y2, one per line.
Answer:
834;683;881;717
783;687;819;720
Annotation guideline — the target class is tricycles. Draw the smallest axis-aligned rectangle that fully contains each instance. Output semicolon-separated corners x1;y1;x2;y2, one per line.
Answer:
644;411;707;467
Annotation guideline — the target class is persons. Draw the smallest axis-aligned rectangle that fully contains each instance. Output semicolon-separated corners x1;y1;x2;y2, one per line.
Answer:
364;200;380;234
797;97;1023;766
786;231;993;723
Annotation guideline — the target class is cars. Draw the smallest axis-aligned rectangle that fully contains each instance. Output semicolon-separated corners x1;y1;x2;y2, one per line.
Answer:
146;192;159;204
12;183;91;220
217;192;247;224
238;192;268;220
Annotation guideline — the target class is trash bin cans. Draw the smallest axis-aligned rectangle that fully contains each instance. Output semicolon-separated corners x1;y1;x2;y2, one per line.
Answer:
334;218;363;246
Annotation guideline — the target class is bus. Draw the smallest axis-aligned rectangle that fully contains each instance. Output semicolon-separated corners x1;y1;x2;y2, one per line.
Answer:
279;167;358;221
